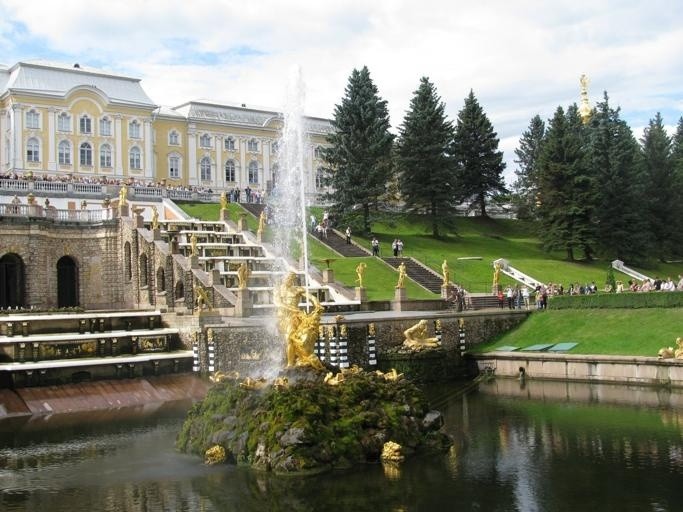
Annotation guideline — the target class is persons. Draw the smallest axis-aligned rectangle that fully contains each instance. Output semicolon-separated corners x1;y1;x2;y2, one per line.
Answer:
392;239;403;258
494;262;500;283
310;211;329;240
231;186;265;203
273;272;321;369
0;171;213;193
498;273;683;311
355;263;367;287
442;260;449;284
346;226;352;244
403;319;439;348
397;262;407;287
371;237;379;256
